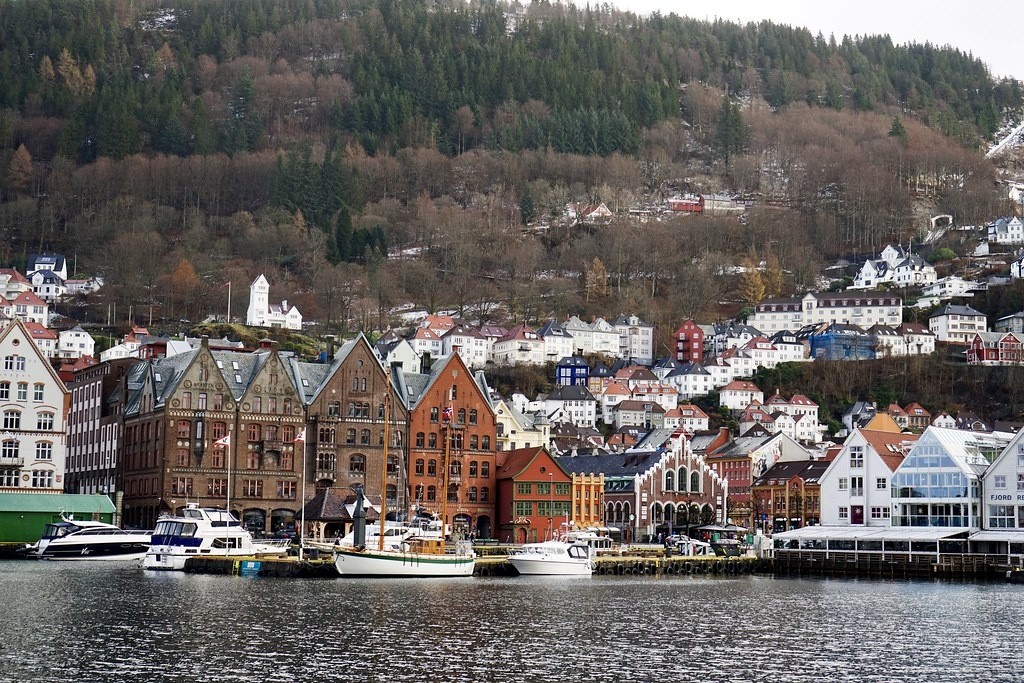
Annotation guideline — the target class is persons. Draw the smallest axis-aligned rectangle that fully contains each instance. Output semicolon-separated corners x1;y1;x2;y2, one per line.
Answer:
648;532;712;544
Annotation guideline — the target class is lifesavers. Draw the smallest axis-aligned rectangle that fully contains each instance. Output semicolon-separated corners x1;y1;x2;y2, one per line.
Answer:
592;559;774;576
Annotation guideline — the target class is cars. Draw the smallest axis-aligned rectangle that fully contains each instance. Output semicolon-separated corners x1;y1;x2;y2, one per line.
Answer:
271;529;298;541
666;534;690;546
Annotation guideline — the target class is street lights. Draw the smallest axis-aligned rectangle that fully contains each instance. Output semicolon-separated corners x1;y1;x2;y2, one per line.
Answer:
548;472;553;541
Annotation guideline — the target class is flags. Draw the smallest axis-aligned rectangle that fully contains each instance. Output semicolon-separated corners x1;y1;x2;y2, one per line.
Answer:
211;435;230;449
291;430;305;443
443;404;453;420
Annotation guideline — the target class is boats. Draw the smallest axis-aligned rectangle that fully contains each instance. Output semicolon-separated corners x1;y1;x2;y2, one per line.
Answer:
505;541;593;575
145;350;475;577
17;521;153;559
